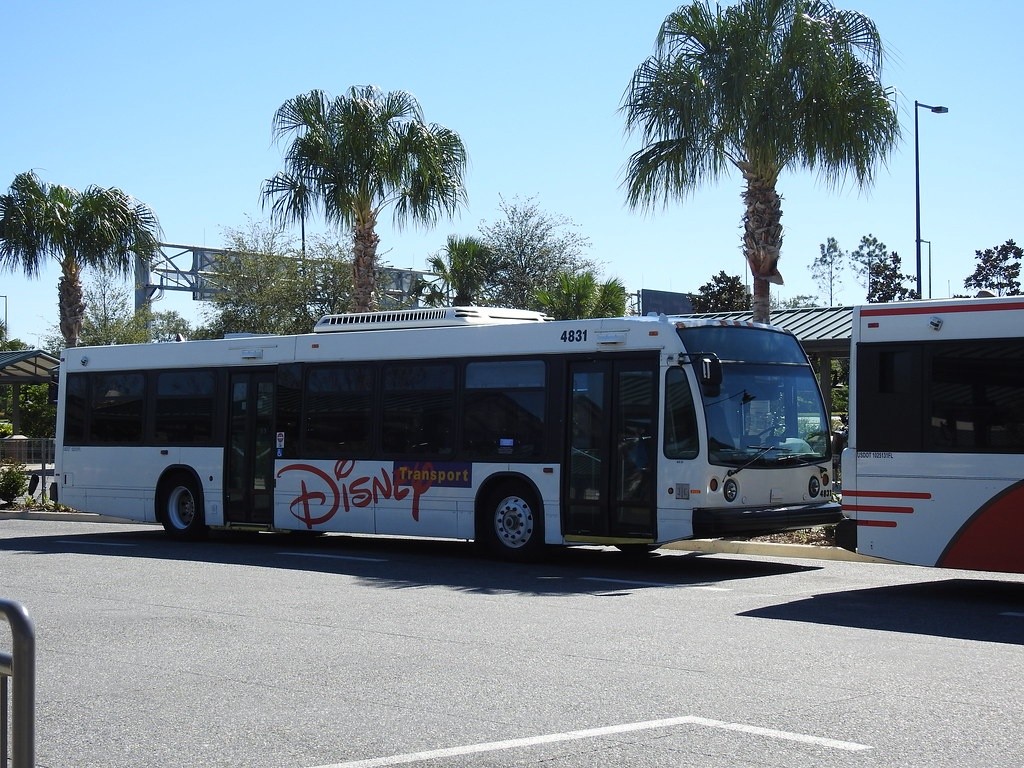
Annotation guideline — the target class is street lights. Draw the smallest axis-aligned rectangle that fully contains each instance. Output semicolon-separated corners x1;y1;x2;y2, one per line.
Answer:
915;99;949;297
915;239;933;299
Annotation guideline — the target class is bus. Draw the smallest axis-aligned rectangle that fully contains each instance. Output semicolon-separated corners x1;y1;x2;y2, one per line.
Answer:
839;296;1024;579
50;307;840;565
